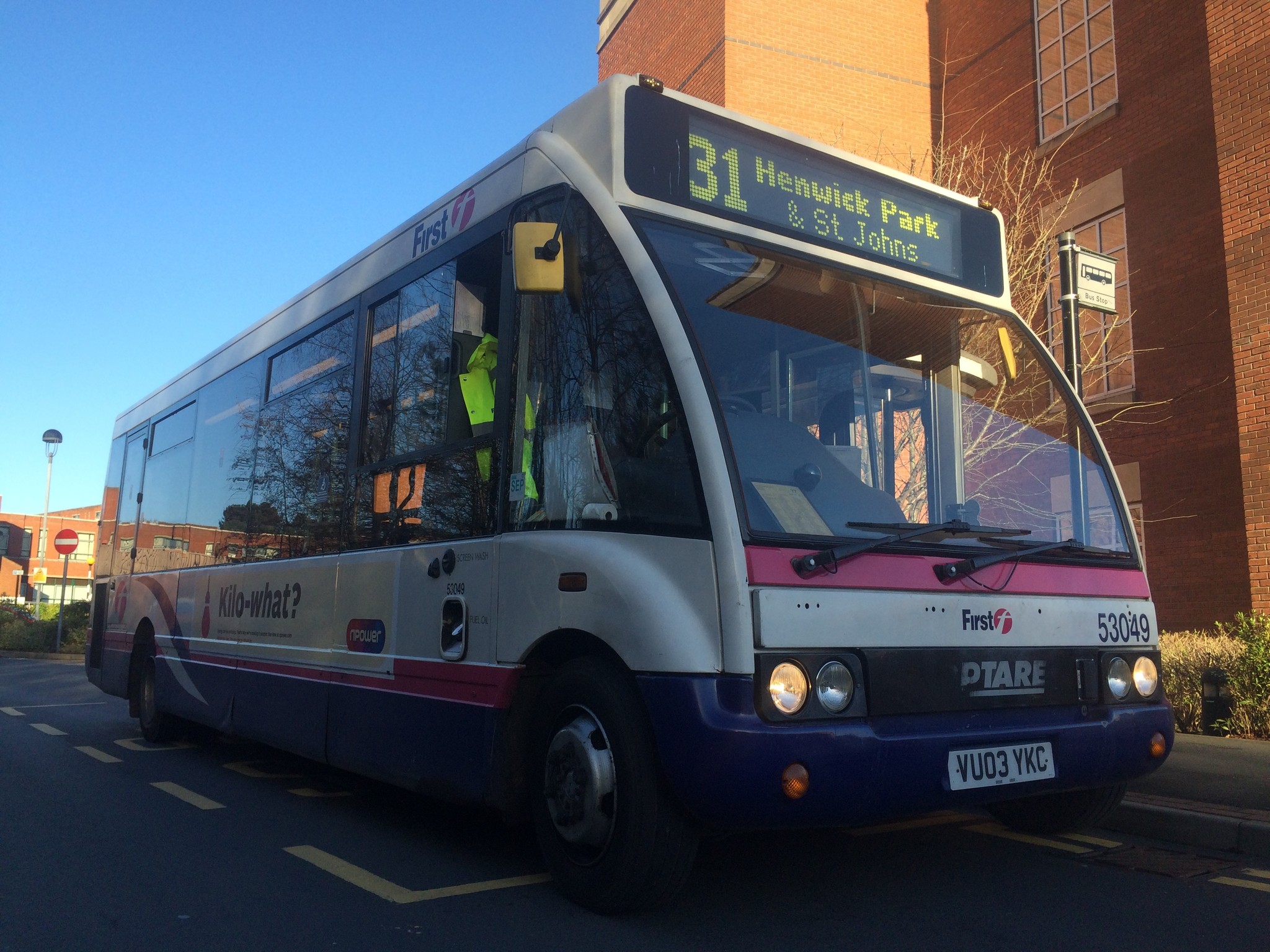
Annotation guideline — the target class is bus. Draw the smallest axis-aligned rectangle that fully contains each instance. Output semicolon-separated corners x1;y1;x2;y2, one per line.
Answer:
84;73;1179;919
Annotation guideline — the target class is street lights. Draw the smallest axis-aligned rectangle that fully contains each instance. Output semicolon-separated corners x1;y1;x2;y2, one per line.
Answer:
35;429;63;620
86;557;94;600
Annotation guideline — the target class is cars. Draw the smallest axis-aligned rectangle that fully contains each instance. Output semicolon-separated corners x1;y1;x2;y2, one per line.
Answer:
0;604;35;622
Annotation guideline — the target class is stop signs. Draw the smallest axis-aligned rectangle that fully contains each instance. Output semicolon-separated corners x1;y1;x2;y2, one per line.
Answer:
54;529;79;555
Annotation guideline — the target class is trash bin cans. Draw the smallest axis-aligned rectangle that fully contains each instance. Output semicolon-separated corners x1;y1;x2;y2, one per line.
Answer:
1199;667;1234;737
